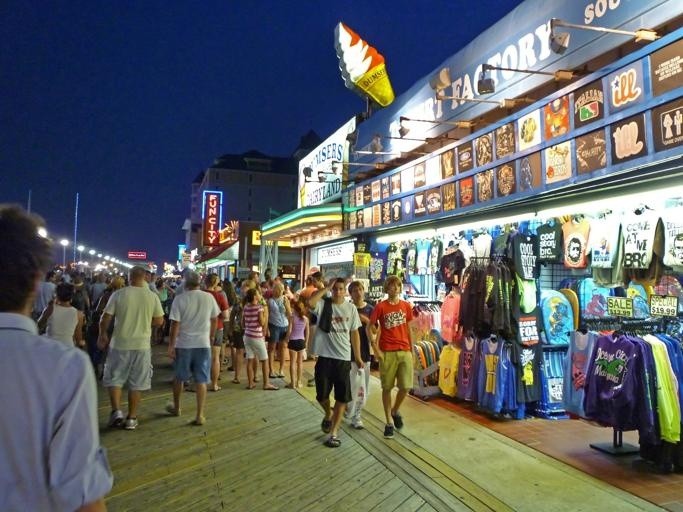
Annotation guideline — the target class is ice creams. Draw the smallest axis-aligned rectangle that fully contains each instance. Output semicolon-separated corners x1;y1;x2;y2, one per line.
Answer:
335;21;395;107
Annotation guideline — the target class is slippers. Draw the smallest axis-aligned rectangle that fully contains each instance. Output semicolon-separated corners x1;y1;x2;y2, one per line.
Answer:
326;436;341;447
321;415;333;433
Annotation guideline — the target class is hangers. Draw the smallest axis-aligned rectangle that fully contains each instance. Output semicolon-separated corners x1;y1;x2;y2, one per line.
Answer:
466;256;515;271
447;343;456;350
513;197;681;239
462;327;508;346
372;220;516;256
409;300;442;320
445;282;460;301
562;313;675;344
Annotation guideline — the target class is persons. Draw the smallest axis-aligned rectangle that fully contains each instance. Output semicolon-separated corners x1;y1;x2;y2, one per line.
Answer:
32;266;414;447
673;233;683;263
0;201;114;512
567;238;581;263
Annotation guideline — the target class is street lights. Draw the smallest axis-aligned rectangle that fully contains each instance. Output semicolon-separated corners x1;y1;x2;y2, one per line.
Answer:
76;244;85;261
58;238;69;266
86;249;134;272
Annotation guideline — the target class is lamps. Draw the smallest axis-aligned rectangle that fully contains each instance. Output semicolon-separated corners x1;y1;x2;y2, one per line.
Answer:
428;66;526;110
395;114;485;139
297;129;438;189
546;16;660;58
476;62;581;94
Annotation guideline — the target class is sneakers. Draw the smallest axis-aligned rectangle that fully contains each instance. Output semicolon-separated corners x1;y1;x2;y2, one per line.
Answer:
106;410;124;428
391;408;402;429
384;424;395;439
350;417;364;430
165;406;181;416
125;415;138;430
